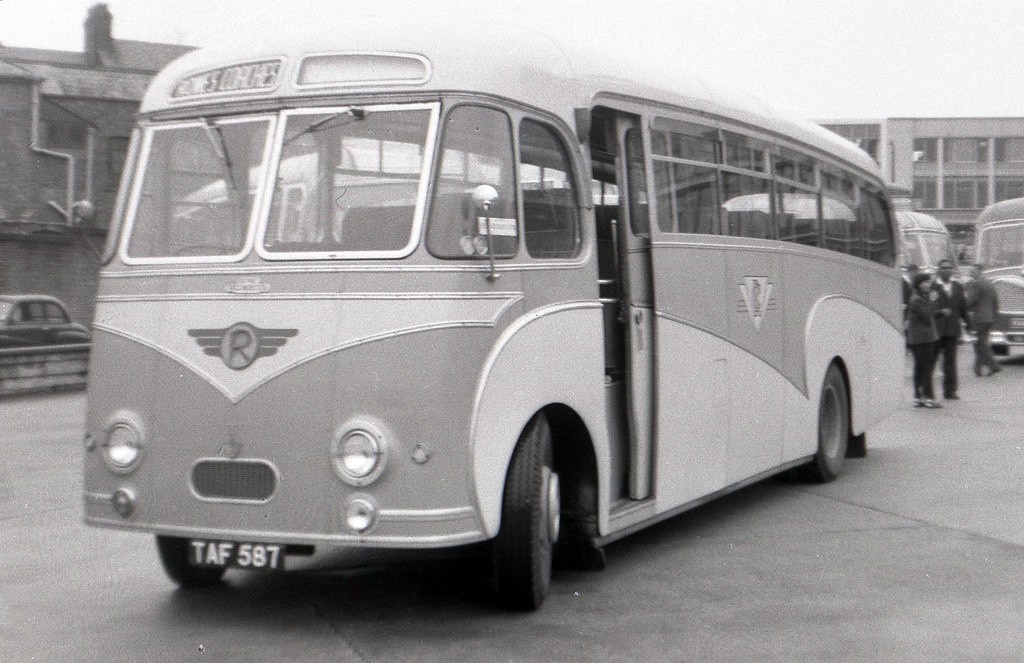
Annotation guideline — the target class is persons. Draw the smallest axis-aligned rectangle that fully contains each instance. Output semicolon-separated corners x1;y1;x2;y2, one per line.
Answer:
901;264;944;409
965;264;1002;378
929;260;976;398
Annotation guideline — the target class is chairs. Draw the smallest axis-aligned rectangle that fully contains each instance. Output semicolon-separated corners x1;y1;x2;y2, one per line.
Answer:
339;187;859;258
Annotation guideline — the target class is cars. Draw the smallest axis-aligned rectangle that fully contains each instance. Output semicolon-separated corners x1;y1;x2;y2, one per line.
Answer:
2;294;89;351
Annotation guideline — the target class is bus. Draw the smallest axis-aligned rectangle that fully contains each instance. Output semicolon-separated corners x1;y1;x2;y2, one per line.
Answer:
80;30;906;609
969;195;1024;366
894;209;964;344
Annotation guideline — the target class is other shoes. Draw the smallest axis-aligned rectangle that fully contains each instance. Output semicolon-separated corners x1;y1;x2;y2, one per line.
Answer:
972;364;982;376
986;366;1001;376
924;401;943;408
943;391;960;400
913;399;926;407
